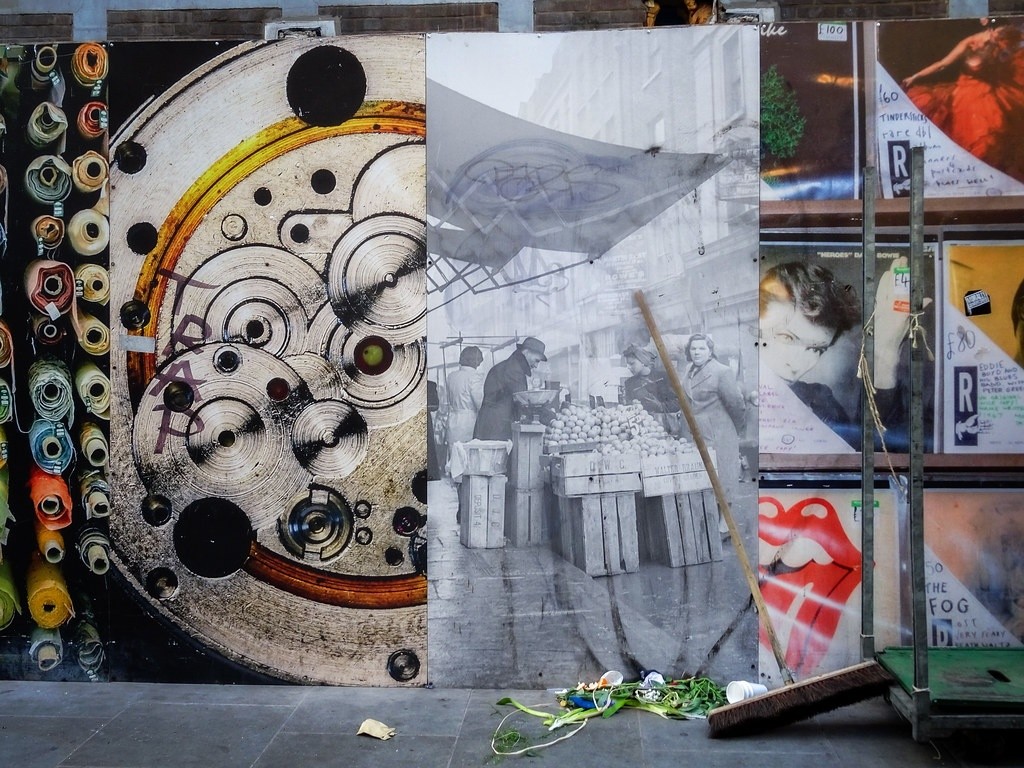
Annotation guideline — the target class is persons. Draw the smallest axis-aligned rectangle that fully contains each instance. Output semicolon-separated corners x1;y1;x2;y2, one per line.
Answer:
446;346;486;525
472;336;559;441
903;15;1024;171
760;263;862;423
680;334;747;543
622;344;680;414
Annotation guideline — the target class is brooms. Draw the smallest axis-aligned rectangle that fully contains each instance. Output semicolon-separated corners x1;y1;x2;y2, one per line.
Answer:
630;288;901;741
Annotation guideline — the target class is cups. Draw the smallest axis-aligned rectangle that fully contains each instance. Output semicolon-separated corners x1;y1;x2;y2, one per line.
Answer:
726;680;769;704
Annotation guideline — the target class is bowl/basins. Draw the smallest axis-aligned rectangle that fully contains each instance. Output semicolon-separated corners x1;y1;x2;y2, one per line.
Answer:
513;388;558;406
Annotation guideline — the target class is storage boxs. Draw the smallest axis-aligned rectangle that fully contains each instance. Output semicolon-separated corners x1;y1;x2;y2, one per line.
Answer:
459;424;718;550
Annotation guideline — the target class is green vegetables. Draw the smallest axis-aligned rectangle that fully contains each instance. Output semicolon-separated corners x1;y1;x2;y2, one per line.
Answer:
496;675;729;731
